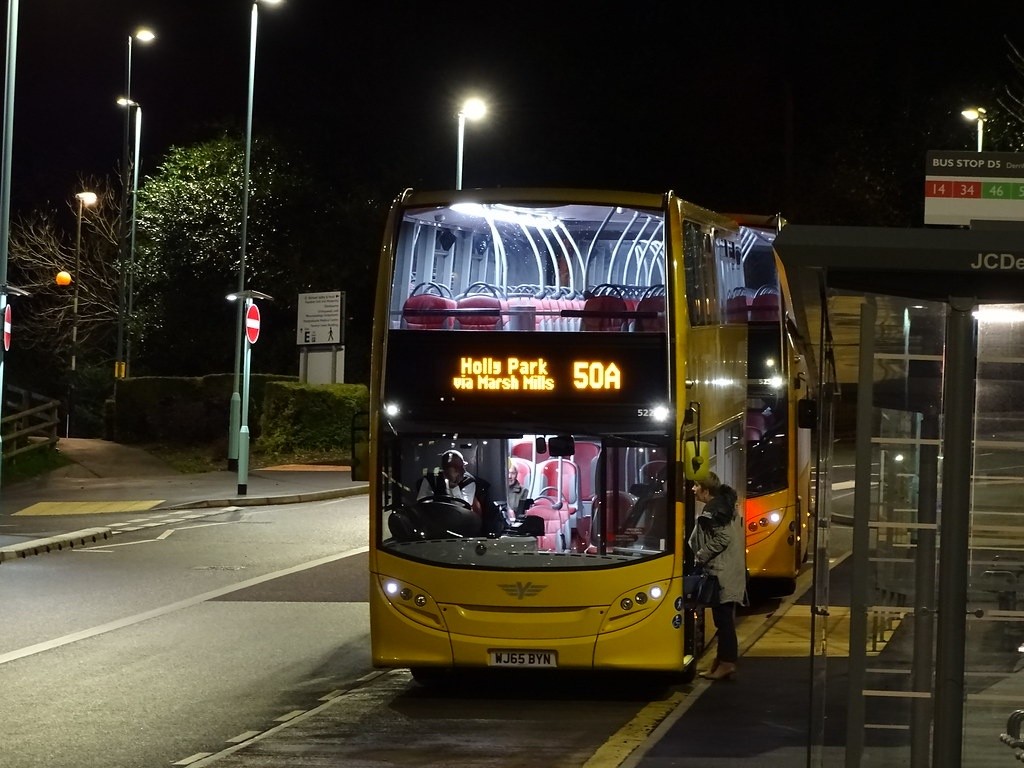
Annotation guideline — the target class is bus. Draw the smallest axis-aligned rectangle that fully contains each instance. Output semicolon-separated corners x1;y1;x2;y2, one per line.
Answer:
347;187;750;692
728;211;818;602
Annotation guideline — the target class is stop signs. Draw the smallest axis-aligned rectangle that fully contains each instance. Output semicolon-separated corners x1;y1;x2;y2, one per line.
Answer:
245;304;261;345
4;304;12;351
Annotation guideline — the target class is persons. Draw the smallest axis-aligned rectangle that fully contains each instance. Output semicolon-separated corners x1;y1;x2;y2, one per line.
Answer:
417;448;476;510
507;464;528;526
688;470;747;682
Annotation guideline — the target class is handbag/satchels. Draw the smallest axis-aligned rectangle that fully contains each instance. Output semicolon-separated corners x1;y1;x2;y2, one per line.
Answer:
682;563;723;609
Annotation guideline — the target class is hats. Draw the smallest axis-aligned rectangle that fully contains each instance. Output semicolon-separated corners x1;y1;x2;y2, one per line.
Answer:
440;450;468;469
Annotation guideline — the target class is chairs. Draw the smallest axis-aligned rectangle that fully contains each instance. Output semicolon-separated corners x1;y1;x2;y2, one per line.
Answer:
747;408;776;442
400;283;665;332
505;440;667;554
722;285;779;321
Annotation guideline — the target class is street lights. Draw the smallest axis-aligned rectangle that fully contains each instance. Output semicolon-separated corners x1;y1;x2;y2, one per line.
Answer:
960;108;987;154
223;0;288;471
112;29;156;415
454;99;484;191
65;192;97;440
114;97;144;376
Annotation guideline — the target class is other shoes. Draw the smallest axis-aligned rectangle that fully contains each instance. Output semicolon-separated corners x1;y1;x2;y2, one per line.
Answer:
699;657;720;678
704;659;738;681
